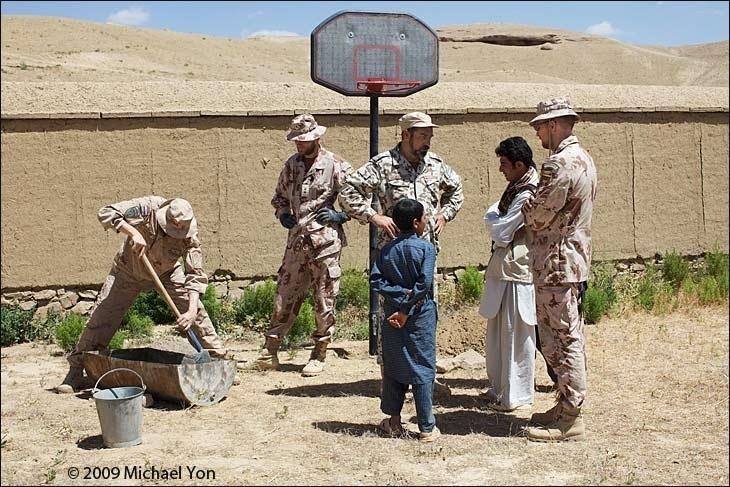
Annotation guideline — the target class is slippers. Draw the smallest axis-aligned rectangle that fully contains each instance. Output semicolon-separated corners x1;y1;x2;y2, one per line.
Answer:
380;417;402;434
419;425;440;442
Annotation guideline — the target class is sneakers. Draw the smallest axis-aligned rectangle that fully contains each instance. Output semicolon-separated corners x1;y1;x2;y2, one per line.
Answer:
480;393;490;401
492;402;534;412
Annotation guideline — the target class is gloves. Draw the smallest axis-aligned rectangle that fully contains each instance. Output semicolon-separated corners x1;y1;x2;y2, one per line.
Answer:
280;213;297;229
315;207;349;225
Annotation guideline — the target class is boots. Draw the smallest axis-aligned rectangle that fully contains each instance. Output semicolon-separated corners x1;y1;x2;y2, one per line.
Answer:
237;336;279;370
301;344;326;376
527;412;585;443
232;374;241;386
532;402;564;424
55;366;84;394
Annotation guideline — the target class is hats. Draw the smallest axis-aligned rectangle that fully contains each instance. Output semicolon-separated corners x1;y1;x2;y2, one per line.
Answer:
398;111;439;131
155;197;199;240
528;97;582;129
286;112;327;143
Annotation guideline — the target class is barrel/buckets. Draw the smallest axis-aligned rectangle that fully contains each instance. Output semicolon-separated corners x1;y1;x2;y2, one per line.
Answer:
91;368;146;448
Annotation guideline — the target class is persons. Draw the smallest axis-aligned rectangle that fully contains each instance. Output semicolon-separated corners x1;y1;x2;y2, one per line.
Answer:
520;96;598;444
53;195;241;394
478;136;541;412
236;114;356;378
370;199;441;443
337;111;452;399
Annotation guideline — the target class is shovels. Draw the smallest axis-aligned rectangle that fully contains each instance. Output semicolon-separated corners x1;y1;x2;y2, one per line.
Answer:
129;239;211;363
